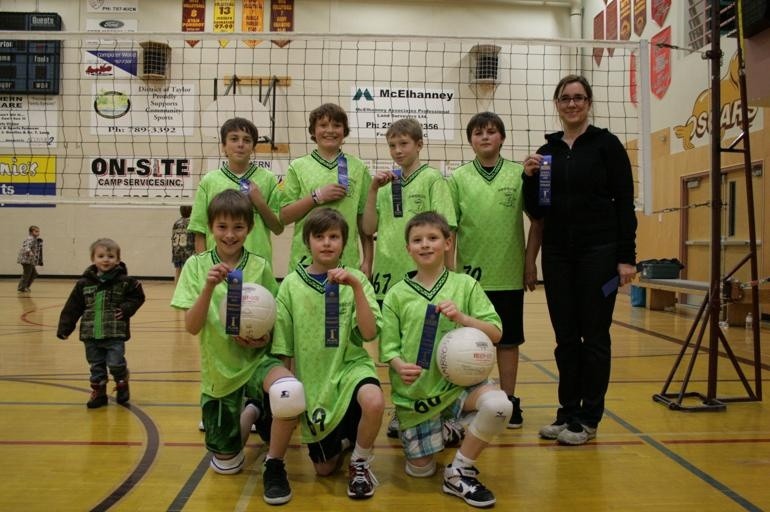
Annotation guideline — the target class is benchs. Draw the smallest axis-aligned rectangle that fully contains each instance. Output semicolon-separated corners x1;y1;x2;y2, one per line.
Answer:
632;278;727;321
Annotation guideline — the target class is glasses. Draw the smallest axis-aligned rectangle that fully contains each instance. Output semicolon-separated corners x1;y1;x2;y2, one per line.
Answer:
554;95;592;105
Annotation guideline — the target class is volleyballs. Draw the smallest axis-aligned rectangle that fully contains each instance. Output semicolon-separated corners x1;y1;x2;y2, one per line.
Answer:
436;326;495;385
219;283;275;340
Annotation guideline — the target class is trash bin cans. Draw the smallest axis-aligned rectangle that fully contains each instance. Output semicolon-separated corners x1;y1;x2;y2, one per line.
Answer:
637;257;685;279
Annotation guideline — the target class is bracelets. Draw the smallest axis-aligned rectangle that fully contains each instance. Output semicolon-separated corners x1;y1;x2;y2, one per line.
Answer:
315;186;323;204
310;189;319;206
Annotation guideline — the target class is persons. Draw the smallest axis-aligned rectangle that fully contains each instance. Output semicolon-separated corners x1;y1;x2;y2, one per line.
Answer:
378;209;515;507
280;102;371;284
444;112;541;430
17;225;44;292
522;75;636;444
187;118;282;433
273;208;384;500
364;120;457;438
57;239;146;407
171;206;193;285
170;188;305;505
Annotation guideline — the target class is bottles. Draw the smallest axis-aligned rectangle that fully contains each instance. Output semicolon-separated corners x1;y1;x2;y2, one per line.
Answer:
745;312;753;345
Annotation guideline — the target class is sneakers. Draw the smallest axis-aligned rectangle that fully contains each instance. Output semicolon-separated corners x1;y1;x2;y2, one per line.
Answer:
25;287;31;293
505;395;525;429
261;456;293;505
17;288;28;294
539;417;568;440
556;419;600;447
441;420;463;449
346;457;375;499
246;400;273;444
115;382;129;403
387;416;404;439
443;463;497;508
87;390;108;409
